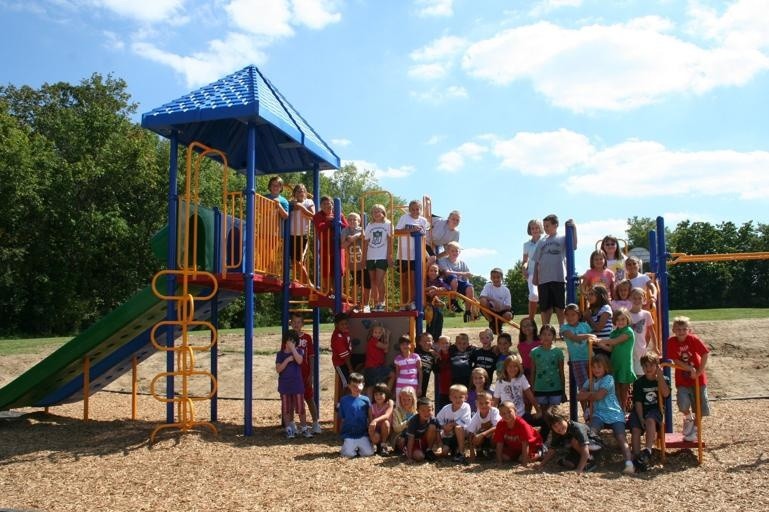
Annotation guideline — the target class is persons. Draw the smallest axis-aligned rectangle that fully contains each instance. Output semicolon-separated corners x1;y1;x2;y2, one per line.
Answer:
312;195;351;301
521;218;545;332
326;235;673;478
275;329;315;439
362;202;395;312
423;209;461;262
664;315;712;443
281;311;323;436
342;211;372;314
394;201;429;312
288;181;316;283
259;175;289;274
530;213;578;341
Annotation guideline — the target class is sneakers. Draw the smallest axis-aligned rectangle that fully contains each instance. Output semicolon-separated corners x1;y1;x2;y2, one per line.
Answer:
447;299;463;312
682;416;698;442
400;301;416;311
426;450;438;464
353;302;386;313
454;450;464;462
585;456;598;472
377;444;389;457
286;421;322;438
464;313;479;322
622;449;651;473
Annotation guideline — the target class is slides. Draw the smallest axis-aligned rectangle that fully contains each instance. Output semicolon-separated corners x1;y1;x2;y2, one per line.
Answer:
1;276;238;409
